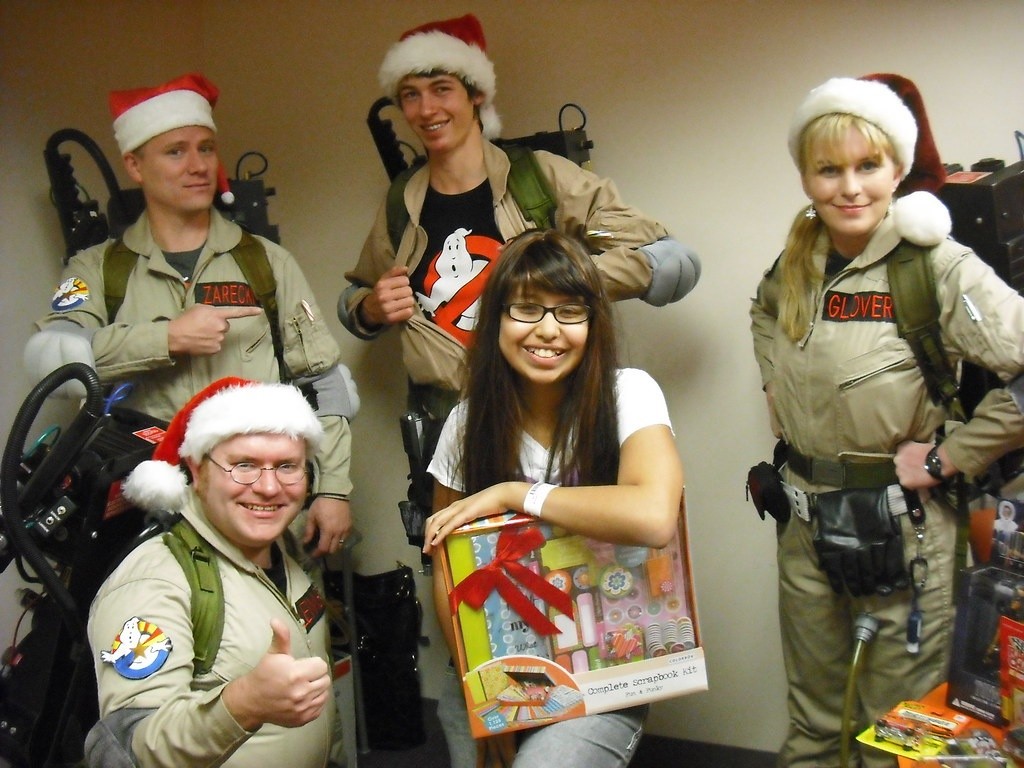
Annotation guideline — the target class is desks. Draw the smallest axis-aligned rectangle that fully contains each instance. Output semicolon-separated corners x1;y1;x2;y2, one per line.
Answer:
898;683;1006;768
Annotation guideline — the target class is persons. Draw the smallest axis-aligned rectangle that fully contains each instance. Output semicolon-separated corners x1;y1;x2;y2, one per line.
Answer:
423;229;683;768
337;9;700;571
23;71;359;658
749;74;1024;768
81;375;337;768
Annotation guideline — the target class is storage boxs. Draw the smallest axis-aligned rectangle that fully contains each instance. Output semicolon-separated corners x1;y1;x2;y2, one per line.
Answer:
331;650;357;768
946;498;1024;733
435;484;711;740
499;666;556;706
465;661;509;704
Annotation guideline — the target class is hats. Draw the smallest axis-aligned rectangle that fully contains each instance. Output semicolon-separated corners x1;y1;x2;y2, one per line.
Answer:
123;375;326;516
378;13;501;140
108;74;235;204
786;72;952;245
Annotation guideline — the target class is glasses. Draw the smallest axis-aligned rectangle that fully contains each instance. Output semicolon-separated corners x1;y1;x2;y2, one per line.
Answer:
204;453;309;486
502;301;594;325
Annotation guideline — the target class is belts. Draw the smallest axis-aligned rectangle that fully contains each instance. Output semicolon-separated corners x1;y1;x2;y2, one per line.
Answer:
776;445;899;489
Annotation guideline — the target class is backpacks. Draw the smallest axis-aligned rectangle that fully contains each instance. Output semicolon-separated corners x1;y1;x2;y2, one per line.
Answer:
886;241;1023;497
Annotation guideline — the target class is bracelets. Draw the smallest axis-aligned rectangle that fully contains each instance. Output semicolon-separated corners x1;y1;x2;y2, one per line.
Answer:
523;481;559;519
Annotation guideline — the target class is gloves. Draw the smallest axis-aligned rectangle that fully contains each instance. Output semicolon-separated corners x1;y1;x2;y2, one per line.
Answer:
810;487;908;599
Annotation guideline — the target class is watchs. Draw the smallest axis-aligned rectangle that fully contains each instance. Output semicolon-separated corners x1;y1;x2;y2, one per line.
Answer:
925;445;945;481
